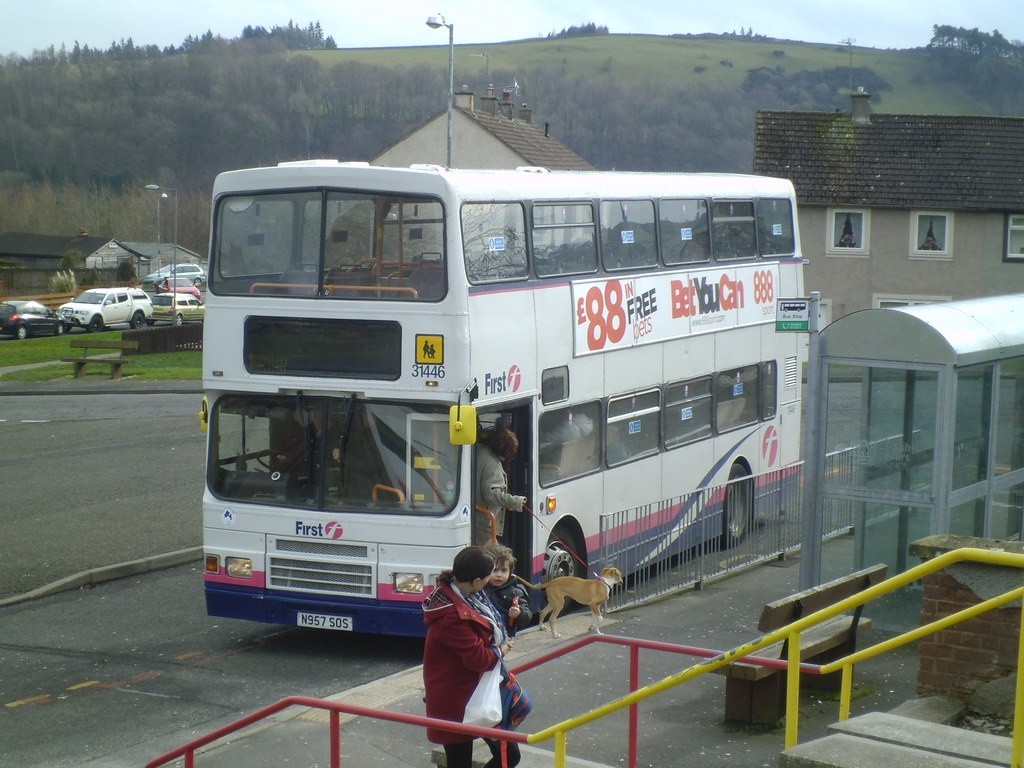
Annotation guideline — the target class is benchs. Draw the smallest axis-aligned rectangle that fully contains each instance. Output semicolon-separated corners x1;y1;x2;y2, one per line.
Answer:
60;340;139;379
709;565;888;732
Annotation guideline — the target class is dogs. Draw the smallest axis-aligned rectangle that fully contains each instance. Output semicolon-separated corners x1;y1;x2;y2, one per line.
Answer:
510;566;624;639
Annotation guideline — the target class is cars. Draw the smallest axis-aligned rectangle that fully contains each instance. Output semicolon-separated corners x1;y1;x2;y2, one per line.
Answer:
0;300;67;340
142;263;207;287
157;278;201;300
146;293;206;326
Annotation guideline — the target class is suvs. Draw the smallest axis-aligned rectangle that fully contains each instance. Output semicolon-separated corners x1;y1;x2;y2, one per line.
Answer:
56;285;154;333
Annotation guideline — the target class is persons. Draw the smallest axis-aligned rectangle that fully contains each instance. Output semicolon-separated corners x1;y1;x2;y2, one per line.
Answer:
536;405;596;448
605;425;632;464
421;546;521;768
441;542;534;643
274;409;346;489
471;426;528;551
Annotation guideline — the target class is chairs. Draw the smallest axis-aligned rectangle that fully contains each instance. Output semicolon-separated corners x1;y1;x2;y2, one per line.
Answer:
542;434;596;480
717;398;745;427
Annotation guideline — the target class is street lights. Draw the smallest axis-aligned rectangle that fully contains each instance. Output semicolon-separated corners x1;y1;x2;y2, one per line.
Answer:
427;10;456;169
145;182;180;328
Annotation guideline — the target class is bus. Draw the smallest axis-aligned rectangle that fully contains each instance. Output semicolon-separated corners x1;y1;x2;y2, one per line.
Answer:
200;158;805;637
